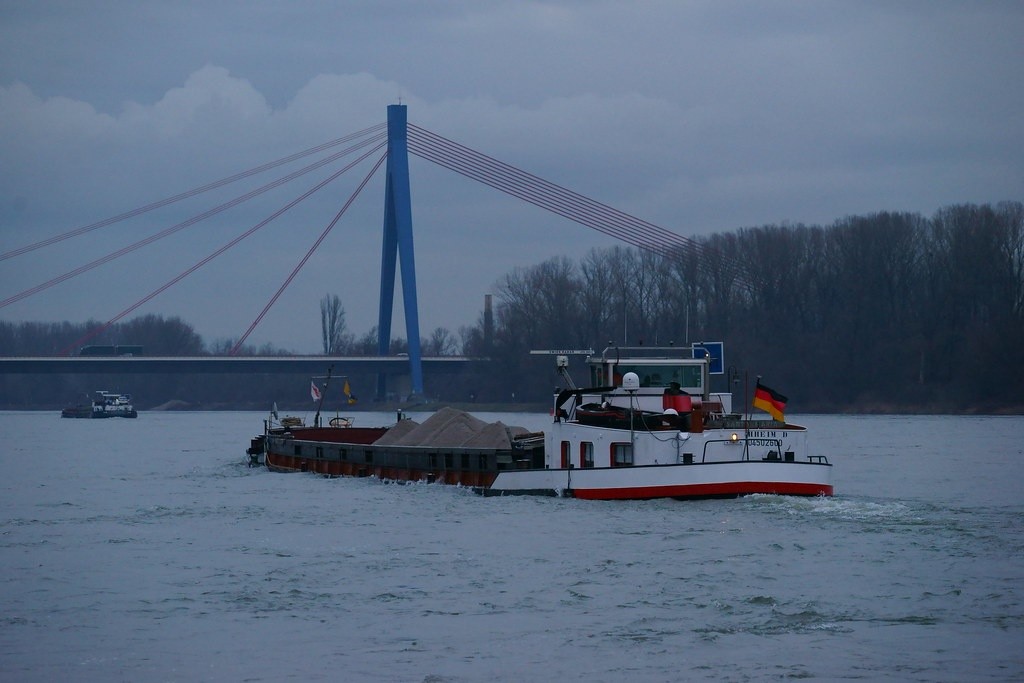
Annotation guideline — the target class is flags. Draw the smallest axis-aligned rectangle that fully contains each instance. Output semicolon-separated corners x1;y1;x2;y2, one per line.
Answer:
753;384;788;423
310;381;321;402
344;377;357;404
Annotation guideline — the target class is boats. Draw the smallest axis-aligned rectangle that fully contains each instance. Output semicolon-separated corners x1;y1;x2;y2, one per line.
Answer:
62;390;138;419
471;305;835;501
245;363;546;489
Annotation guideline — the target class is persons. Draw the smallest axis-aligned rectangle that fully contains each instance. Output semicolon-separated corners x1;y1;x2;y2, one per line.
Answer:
597;366;622;387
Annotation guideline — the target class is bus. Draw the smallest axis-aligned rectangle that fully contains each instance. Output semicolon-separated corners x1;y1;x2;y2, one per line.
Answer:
79;344;140;358
